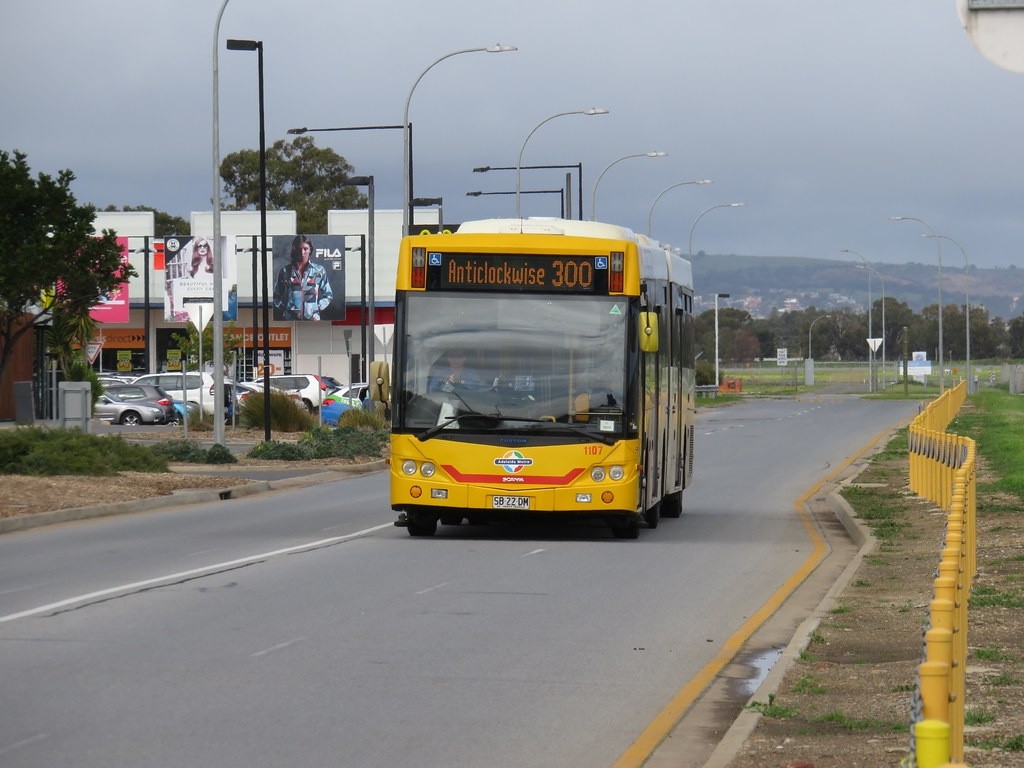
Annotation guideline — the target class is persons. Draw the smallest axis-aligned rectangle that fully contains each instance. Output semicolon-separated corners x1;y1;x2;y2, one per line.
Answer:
273;236;333;321
187;238;214;279
428;343;488;395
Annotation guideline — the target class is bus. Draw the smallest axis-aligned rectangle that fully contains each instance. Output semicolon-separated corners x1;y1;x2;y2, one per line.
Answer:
386;214;697;542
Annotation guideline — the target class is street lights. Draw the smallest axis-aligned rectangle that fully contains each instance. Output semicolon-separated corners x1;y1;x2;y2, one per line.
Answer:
339;176;375;363
516;107;609;218
714;293;730;386
591;151;746;256
286;122;415;227
465;163;583;221
402;45;518;236
226;39;271;445
803;215;971;396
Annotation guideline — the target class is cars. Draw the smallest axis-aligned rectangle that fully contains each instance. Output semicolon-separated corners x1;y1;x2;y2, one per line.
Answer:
92;371;392;426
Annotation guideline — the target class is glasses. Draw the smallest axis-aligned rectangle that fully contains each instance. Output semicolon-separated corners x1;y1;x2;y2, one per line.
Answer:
197;243;208;249
448;353;466;359
